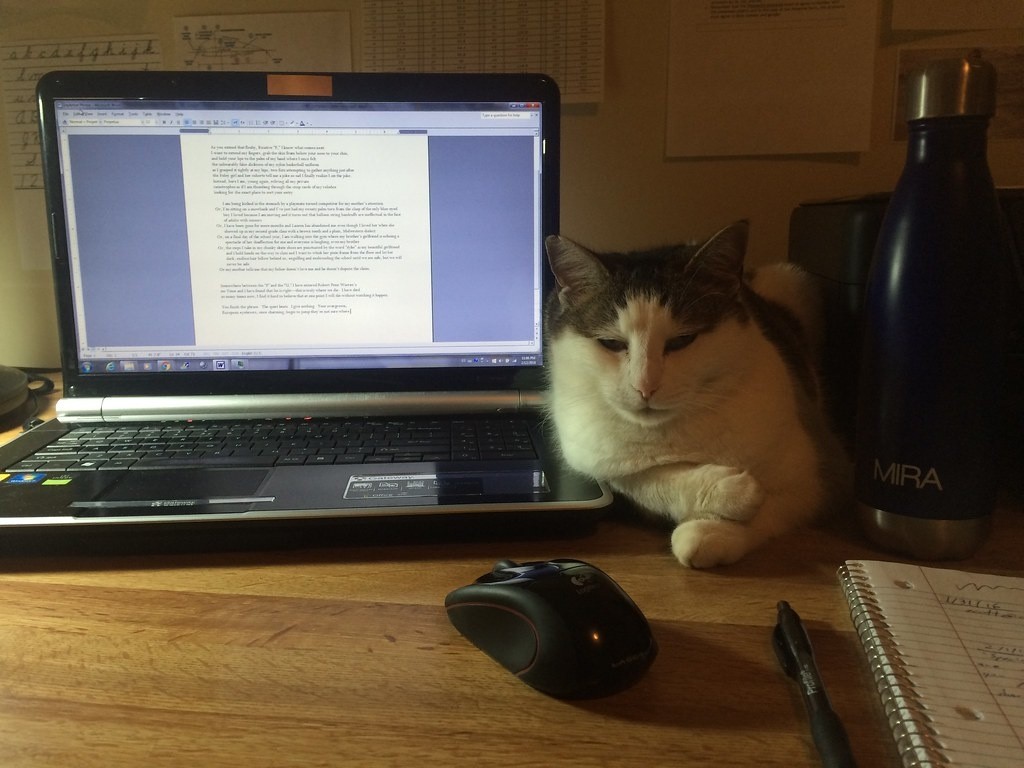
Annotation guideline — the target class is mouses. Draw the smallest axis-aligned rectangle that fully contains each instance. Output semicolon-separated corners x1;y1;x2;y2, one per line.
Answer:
446;560;657;701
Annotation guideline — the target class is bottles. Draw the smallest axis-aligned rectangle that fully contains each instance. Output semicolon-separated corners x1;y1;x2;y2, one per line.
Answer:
847;58;1008;555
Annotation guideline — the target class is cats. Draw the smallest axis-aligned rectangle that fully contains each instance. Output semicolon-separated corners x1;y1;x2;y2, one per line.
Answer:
545;219;833;570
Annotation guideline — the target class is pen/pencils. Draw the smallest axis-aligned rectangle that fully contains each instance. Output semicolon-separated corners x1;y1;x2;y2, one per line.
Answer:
772;599;861;768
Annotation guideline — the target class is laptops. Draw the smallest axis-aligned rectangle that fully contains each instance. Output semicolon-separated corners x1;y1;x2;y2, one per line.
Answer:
0;69;612;539
